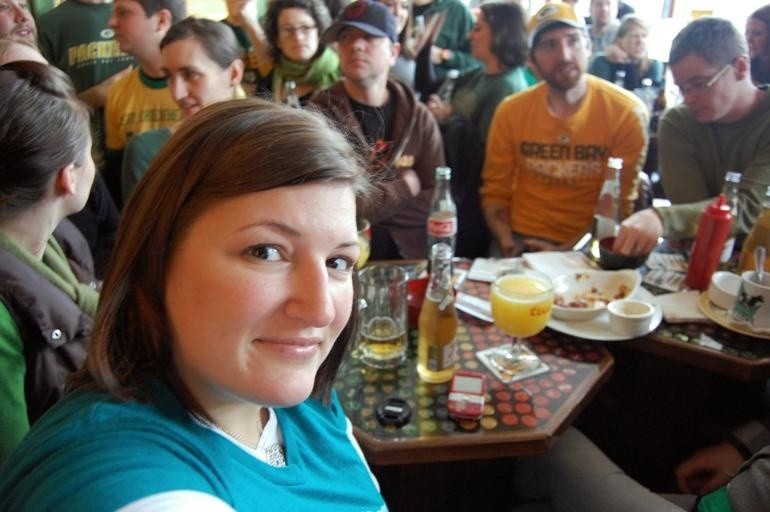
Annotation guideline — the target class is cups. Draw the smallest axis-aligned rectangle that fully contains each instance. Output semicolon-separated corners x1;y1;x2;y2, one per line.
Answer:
608;299;654;334
730;271;770;332
358;264;408;360
707;271;742;308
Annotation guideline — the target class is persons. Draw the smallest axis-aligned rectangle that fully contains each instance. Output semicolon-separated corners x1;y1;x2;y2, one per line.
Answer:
612;17;770;259
744;4;770;90
480;3;651;258
2;1;665;213
0;61;102;468
303;0;447;260
513;412;769;511
0;98;388;512
1;36;121;293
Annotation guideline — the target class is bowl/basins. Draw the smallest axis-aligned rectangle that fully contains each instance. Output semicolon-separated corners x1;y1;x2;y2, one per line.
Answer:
599;236;650;269
549;269;641;322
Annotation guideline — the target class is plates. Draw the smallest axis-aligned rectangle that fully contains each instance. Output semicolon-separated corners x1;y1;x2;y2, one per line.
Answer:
546;287;665;341
698;291;770;339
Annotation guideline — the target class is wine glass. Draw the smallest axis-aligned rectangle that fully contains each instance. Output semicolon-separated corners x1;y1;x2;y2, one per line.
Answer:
490;269;555;375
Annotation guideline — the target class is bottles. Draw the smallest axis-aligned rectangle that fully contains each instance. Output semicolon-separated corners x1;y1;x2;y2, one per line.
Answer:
614;71;625;87
737;185;770;271
426;167;458;275
417;243;457;384
284;82;299;110
649;88;668;130
718;172;742;269
685;193;734;289
416;15;425;35
590;157;623;239
437;69;459;128
642;78;653;108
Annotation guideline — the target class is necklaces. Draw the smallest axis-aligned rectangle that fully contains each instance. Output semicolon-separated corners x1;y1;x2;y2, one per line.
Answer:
211;409;264;448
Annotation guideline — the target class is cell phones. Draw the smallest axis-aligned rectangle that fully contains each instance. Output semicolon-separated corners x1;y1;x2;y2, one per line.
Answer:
445;370;486;419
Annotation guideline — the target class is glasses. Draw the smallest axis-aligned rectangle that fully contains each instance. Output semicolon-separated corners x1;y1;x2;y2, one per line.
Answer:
279;20;315;35
676;60;733;95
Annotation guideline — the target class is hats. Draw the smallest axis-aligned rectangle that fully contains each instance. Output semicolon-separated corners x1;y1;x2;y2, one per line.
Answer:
323;0;397;45
527;1;586;49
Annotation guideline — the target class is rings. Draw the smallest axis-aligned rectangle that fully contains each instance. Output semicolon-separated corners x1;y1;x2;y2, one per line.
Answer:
637;244;643;253
